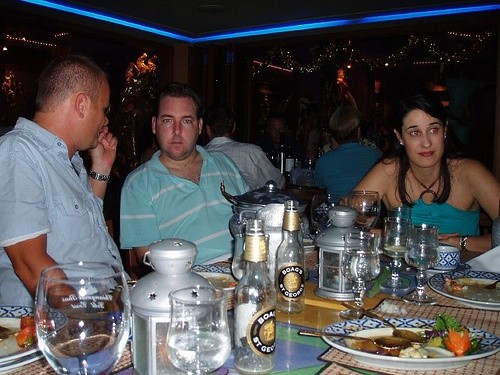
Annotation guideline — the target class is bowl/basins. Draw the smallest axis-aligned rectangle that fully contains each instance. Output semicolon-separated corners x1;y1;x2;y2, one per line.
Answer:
430;245;461;273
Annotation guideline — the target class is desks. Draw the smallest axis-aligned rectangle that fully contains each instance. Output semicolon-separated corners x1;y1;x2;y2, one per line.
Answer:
290;186;326;200
7;252;500;375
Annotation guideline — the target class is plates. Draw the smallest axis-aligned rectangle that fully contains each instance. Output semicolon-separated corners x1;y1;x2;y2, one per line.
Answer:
322;316;500;370
428;271;500;310
190;265;237;291
0;306;67;371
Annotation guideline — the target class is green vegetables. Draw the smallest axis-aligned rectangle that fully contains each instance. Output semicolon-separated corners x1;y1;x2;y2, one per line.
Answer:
419;313;482;358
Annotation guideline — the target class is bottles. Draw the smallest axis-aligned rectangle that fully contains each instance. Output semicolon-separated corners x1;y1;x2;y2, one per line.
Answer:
274;201;307;313
232;218;276;375
284;138;312;173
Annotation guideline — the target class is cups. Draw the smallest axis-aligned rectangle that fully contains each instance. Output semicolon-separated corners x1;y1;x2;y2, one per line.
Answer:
35;261;131;375
308;194;337;230
348;190;379;230
166;286;231;375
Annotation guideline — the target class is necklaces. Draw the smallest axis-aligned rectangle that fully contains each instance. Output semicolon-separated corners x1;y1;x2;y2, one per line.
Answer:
409;167;441;202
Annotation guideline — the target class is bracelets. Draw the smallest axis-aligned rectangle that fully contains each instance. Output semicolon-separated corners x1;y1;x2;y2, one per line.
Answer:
87;170;111;181
460;235;467;251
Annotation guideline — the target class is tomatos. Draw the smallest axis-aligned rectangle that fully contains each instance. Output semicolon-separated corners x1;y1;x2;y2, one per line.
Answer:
15;310;54;347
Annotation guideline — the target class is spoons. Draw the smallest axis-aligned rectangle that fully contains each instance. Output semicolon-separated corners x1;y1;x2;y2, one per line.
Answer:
297;304;420;350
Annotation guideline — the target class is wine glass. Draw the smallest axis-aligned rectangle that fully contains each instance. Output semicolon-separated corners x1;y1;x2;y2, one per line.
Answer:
378;218;412;291
341;230;380;319
404;224;441;305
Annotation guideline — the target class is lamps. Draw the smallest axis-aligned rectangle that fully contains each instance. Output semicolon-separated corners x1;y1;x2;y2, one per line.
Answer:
128;238;212;375
314;205;374;300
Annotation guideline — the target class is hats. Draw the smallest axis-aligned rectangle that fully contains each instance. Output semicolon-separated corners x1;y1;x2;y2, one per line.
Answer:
329;104;362;132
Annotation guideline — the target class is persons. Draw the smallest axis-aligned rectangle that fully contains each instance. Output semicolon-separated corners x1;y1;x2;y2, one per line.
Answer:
339;90;500;252
205;105;382;205
0;55;132;315
120;82;252;268
